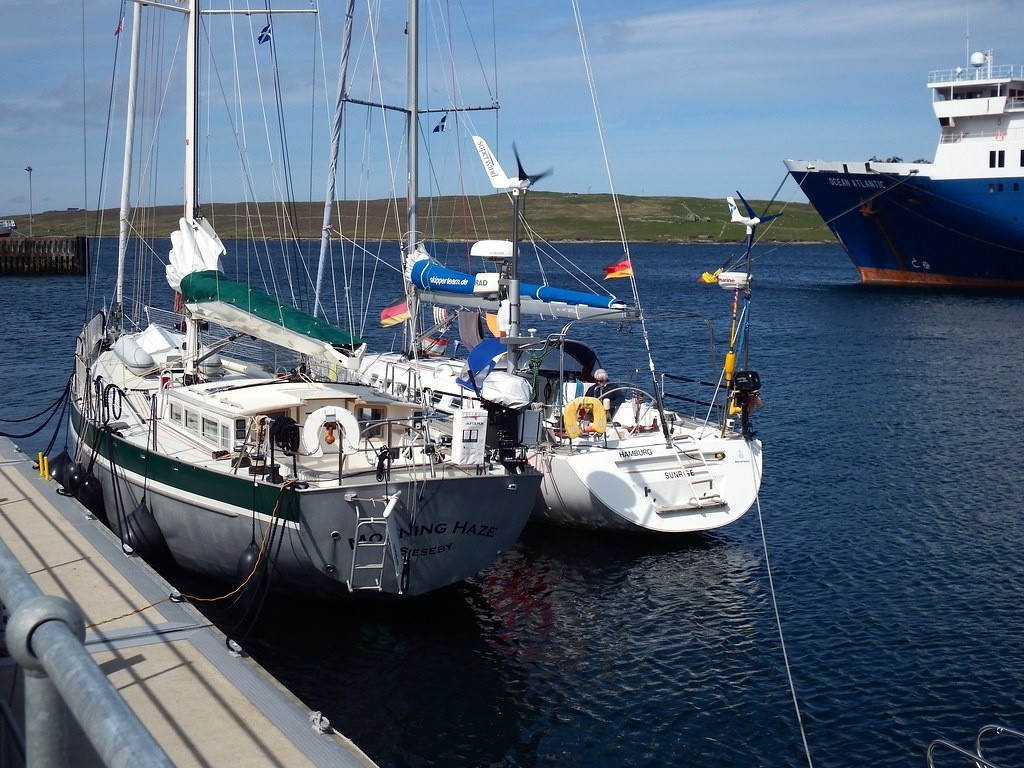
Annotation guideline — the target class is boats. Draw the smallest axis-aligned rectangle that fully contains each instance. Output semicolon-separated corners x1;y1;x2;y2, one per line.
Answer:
780;14;1024;290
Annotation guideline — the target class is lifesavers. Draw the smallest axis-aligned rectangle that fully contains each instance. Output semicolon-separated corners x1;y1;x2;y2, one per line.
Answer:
996;134;1004;142
564;396;607;440
301;405;360;459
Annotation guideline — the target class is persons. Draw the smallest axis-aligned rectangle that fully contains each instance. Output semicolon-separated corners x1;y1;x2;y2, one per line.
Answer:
585;369;625;422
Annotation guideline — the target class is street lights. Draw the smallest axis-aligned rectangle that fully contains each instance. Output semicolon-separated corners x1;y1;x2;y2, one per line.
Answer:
25;166;33;237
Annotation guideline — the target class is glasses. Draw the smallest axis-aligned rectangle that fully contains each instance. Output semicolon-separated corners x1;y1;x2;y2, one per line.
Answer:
596;380;604;383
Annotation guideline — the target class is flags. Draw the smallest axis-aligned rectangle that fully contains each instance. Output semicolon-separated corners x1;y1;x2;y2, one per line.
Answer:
433;307;450;333
603;253;634;280
380;294;412;328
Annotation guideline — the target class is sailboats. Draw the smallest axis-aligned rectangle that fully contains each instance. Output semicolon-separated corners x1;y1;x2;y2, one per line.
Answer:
277;0;766;542
58;1;547;601
